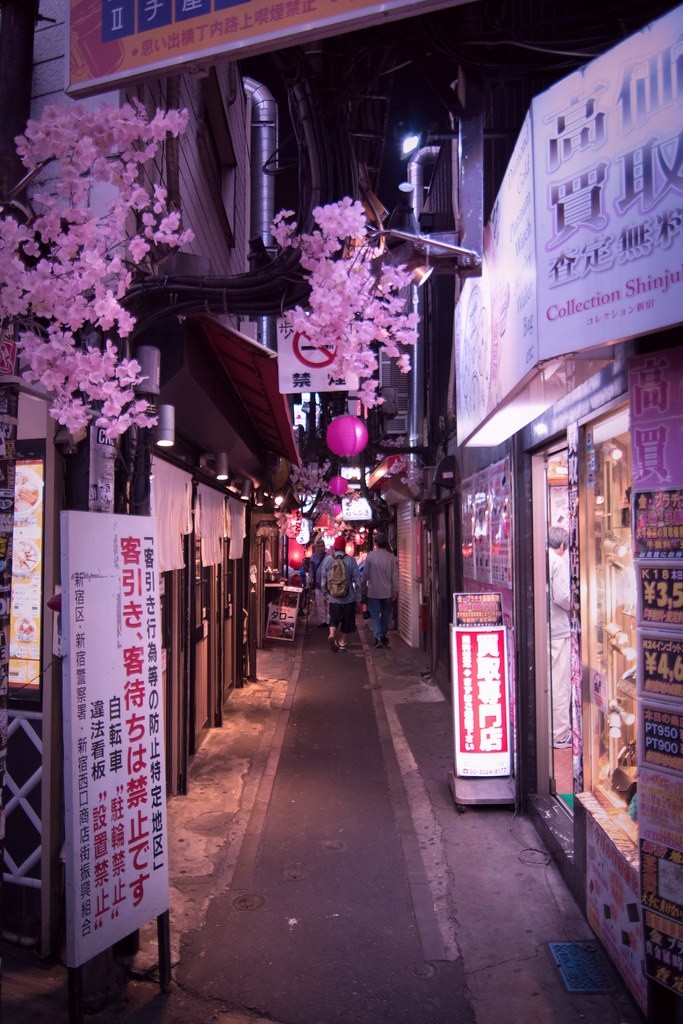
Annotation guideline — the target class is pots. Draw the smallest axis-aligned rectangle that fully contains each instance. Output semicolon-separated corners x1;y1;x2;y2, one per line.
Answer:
264;571;282;583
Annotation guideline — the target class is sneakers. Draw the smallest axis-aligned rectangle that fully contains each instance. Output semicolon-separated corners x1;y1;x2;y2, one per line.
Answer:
553;730;572;748
328;635;339;651
339;640;351;650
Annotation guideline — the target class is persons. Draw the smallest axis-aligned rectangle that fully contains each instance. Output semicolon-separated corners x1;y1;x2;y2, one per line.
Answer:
546;526;574;749
298;533;399;653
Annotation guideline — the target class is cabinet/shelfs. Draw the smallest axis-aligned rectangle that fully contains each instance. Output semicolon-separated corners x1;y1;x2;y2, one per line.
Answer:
591;525;639;848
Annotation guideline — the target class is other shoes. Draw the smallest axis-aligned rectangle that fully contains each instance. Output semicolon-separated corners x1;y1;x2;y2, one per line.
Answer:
383;640;390;647
317;622;328;627
374;639;383;648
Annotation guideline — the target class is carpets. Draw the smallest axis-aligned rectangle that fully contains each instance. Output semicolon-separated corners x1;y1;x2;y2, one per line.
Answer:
558;793;573;811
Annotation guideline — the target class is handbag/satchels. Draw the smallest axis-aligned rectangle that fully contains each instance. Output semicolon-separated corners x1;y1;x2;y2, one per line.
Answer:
611;767;638;806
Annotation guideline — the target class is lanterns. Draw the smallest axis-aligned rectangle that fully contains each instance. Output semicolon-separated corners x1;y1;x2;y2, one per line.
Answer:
326;414;369;457
285;504;366;570
328;475;349;497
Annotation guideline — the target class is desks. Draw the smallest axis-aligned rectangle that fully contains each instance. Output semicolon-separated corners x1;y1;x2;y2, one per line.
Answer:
264;581;285;621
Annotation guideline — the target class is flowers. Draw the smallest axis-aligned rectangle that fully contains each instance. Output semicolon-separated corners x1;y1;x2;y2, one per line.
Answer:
0;95;195;443
270;197;422;410
376;435;425;485
272;460;362;539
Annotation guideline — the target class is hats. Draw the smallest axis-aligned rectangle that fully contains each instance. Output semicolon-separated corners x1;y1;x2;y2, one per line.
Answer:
334;537;346;549
375;533;387;545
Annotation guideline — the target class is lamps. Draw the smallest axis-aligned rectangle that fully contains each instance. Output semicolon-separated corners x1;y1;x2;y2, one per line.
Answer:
206;450;229;480
252;489;264;506
404;254;433;288
155;404;174;445
609;639;636;661
600;536;631;558
135;345;160;395
607;700;635;725
236;480;251;500
400;129;458;161
608;711;621;738
605;624;628;644
600;443;622;460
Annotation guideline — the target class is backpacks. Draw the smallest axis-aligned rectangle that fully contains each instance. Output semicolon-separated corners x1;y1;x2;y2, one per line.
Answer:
325;554;352;598
